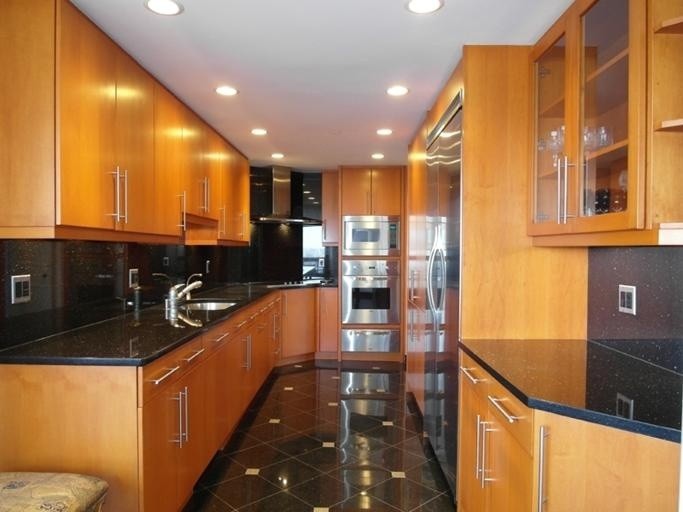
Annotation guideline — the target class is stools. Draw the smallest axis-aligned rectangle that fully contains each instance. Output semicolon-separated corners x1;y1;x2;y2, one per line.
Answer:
0;471;109;512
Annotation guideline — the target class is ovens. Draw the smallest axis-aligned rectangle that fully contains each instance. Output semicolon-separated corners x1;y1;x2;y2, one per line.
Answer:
341;276;399;323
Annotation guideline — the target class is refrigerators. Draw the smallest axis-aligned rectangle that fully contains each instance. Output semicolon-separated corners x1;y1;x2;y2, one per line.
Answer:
424;108;458;507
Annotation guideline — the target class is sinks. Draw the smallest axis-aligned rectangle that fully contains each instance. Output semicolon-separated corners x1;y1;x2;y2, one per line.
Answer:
178;298;242;311
122;299;158;305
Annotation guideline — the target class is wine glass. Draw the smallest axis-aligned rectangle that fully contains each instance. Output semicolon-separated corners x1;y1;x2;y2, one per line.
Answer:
580;185;626;215
550;122;612;167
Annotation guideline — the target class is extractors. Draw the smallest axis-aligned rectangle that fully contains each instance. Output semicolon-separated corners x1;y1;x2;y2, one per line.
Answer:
249;164;321;226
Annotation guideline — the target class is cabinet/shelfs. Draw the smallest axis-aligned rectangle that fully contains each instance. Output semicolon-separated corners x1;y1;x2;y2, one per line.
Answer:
455;348;534;512
206;310;255;466
321;170;340;246
405;120;425;436
255;297;280;394
529;0;646;247
338;164;403;214
154;77;183;243
0;0;154;243
646;0;683;246
282;290;315;365
2;337;206;511
316;287;339;359
183;103;218;226
182;133;250;247
534;409;681;512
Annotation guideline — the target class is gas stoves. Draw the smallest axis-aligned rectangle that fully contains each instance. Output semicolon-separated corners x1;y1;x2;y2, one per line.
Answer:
248;279;315;288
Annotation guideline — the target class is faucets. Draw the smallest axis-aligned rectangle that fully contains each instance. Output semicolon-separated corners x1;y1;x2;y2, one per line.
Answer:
185;273;204;299
133;285;143;311
164;281;202;320
151;272;174;287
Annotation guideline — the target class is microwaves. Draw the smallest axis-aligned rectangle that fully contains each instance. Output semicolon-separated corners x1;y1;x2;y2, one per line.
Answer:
344;221;399;250
